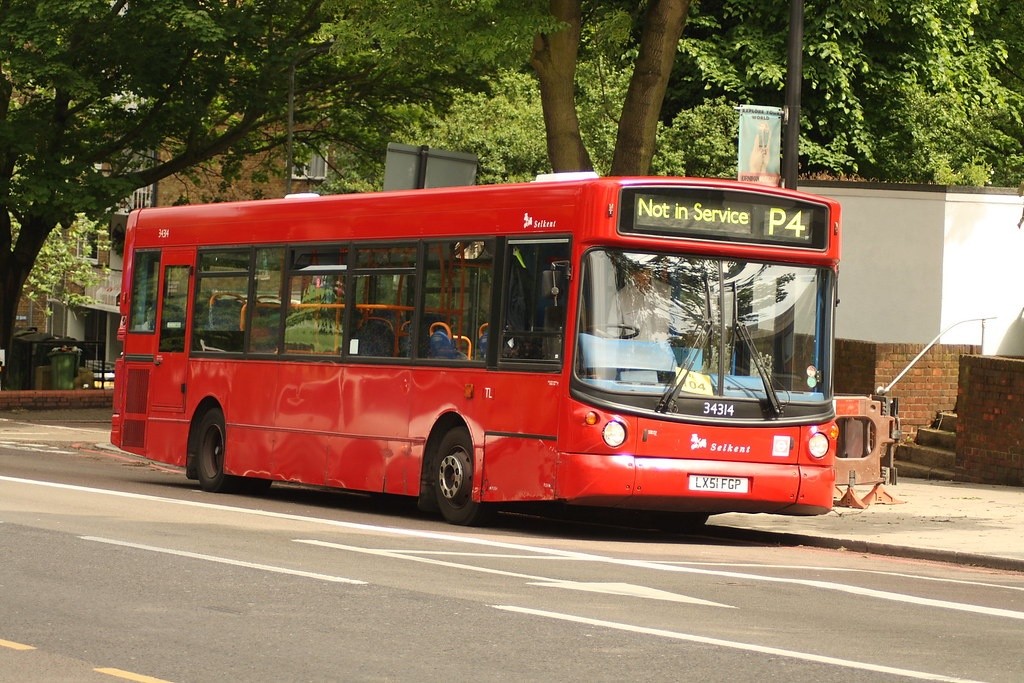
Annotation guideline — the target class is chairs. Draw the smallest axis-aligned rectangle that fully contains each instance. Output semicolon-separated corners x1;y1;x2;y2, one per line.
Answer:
144;293;488;360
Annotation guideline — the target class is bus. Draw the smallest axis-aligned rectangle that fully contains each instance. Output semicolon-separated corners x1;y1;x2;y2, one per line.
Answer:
110;169;844;523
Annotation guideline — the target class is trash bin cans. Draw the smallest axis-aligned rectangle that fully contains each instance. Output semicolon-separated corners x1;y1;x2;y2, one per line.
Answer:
49;345;83;390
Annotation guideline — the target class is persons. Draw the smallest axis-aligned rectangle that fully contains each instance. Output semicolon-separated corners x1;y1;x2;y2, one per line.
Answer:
354;309;411;357
363;277;378;323
422;315;468;359
606;262;672;383
